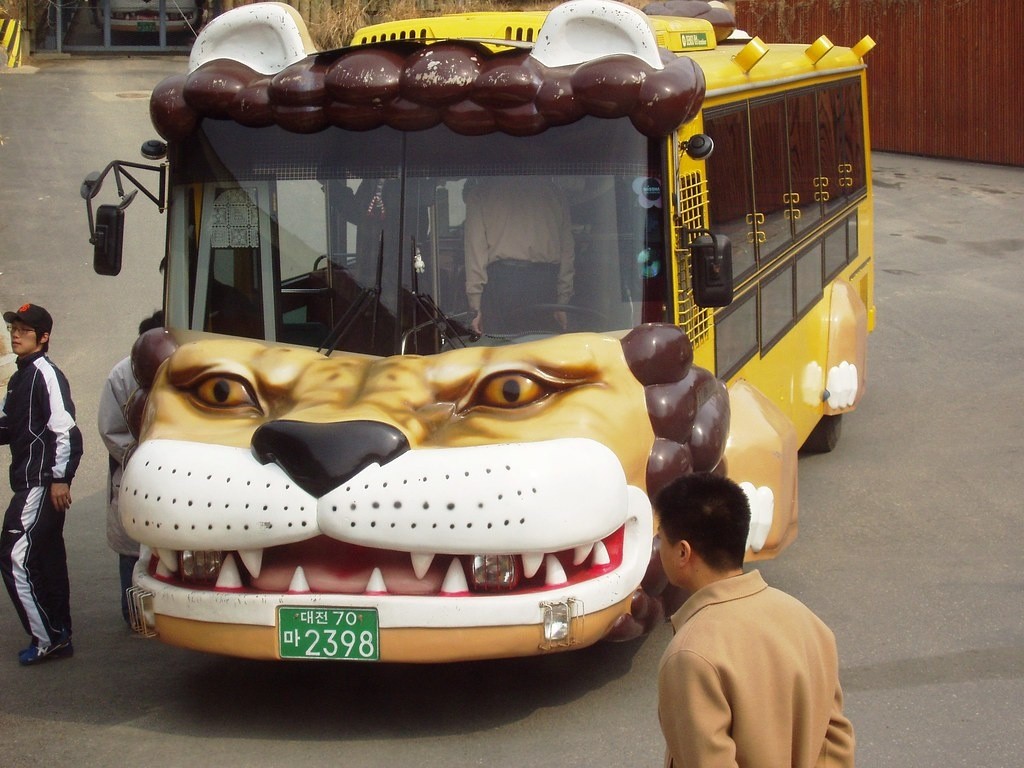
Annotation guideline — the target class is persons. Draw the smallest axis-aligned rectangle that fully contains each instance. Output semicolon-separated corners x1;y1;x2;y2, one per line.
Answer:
0;303;83;663
318;169;429;313
98;309;163;626
458;175;632;338
655;471;855;768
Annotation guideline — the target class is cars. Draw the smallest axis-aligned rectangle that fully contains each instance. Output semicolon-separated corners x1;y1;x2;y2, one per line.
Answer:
94;1;197;36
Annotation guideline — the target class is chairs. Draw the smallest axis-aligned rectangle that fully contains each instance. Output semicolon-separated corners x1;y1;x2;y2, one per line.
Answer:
305;253;360;349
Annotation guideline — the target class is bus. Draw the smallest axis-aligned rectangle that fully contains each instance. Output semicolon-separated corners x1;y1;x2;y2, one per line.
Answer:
81;2;874;664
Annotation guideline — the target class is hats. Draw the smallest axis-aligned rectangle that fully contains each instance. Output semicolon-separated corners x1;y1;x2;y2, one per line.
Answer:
3;303;53;333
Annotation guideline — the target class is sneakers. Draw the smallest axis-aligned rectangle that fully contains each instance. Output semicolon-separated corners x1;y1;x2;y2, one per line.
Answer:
18;638;72;665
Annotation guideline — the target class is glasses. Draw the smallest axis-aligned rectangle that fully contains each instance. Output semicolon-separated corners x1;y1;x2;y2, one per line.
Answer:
7;325;38;336
